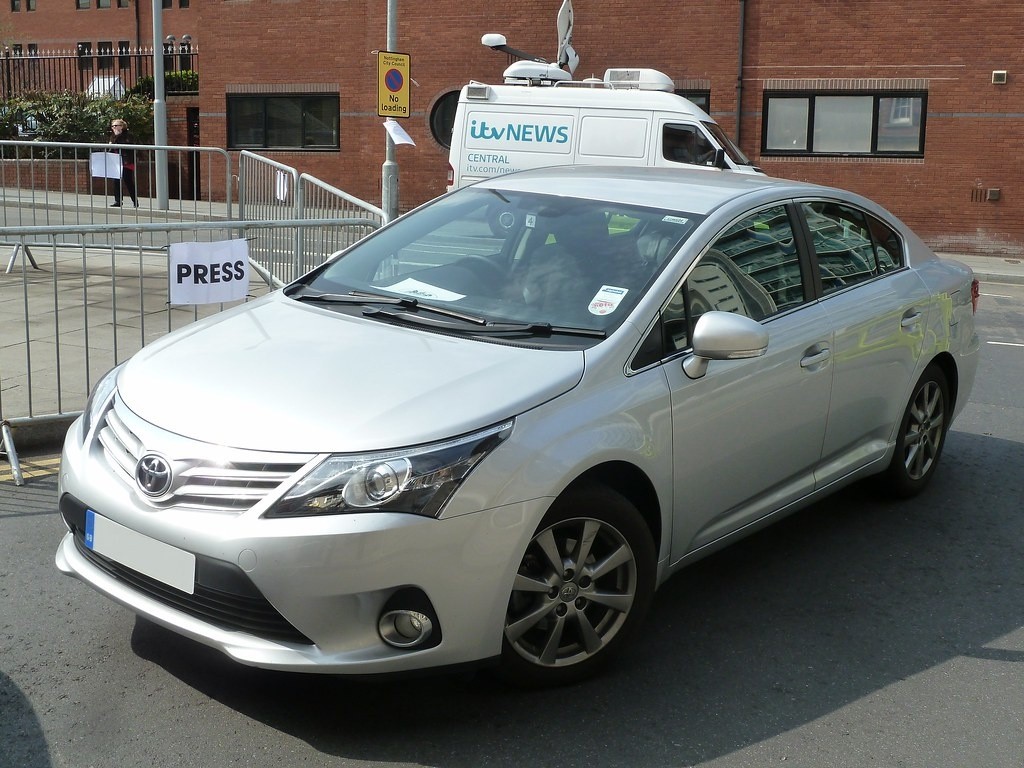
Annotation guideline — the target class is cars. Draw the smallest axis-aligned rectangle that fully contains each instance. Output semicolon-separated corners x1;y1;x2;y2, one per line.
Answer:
53;161;985;693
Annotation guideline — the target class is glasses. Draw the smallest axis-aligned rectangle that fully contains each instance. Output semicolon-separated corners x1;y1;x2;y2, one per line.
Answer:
112;124;122;127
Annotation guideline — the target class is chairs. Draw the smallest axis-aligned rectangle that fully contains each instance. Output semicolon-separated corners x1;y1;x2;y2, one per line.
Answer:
523;206;619;318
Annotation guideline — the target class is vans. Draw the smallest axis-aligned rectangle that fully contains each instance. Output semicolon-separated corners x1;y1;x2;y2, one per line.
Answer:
438;0;770;195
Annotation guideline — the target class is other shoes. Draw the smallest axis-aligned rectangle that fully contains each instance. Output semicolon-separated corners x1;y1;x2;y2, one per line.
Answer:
134;202;139;208
110;201;123;207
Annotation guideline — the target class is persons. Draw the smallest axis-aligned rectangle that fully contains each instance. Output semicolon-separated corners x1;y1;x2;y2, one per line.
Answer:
107;118;140;208
